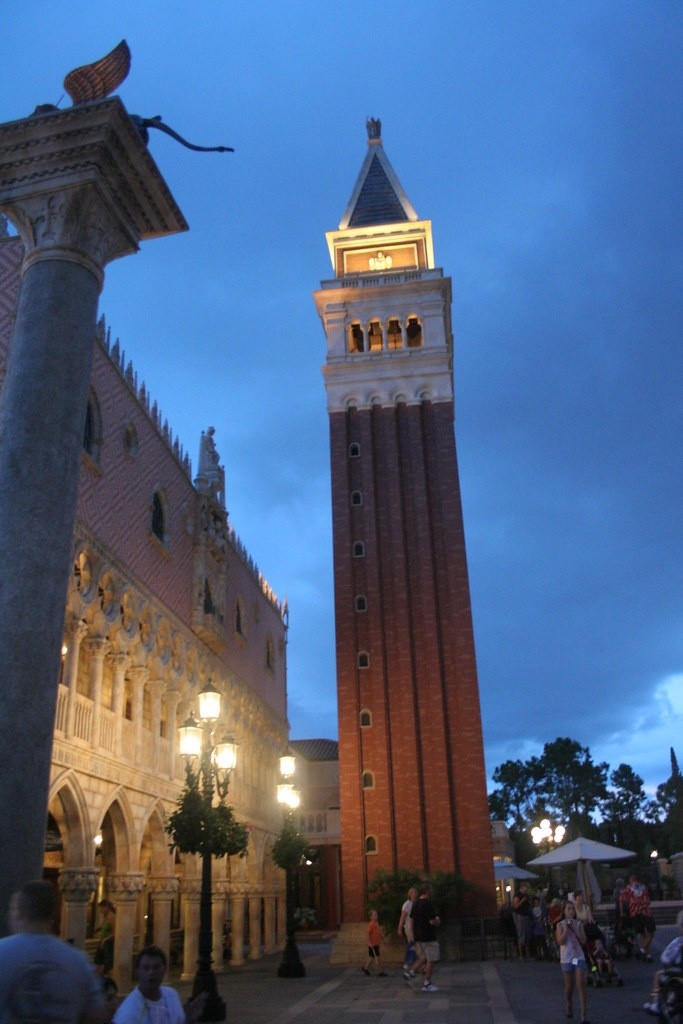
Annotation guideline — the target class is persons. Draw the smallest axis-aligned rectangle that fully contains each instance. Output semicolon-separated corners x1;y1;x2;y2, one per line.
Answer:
397;885;441;993
555;900;590;1024
92;896;116;978
181;968;227;1024
361;908;387;977
586;939;613;975
513;882;530;961
614;875;657;962
0;880;109;1024
641;910;683;1012
570;889;596;924
529;882;574;963
111;945;186;1024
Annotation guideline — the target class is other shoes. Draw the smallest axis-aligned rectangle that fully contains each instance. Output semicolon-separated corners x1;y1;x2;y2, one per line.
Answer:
376;971;387;976
564;1003;573;1018
422;982;440;992
403;970;416;980
643;1002;658;1012
362;966;370;975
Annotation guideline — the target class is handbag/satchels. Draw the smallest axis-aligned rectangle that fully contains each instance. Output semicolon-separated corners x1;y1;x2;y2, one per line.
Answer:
582;945;588;958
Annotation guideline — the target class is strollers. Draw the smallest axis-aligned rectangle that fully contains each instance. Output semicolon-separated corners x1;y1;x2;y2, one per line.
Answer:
581;922;626;989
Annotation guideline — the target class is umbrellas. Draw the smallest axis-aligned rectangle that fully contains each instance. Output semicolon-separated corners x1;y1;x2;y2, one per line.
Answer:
493;860;539;906
526;837;637;913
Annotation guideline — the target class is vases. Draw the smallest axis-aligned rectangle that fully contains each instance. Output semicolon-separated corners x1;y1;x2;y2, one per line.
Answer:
300;919;311;930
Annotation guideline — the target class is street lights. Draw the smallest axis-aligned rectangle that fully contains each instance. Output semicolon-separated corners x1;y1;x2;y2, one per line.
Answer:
273;744;307;979
531;818;566;889
176;677;239;1008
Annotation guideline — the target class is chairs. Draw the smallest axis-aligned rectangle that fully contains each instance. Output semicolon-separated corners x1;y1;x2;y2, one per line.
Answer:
458;917;484;962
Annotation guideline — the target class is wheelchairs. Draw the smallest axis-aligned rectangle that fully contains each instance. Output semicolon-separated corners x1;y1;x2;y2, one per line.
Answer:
651;947;683;1024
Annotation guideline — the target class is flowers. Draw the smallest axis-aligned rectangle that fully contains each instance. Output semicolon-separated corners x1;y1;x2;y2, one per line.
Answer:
293;906;318;922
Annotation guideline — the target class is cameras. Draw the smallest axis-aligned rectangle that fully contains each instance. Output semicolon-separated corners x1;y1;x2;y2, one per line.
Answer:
567;919;573;925
